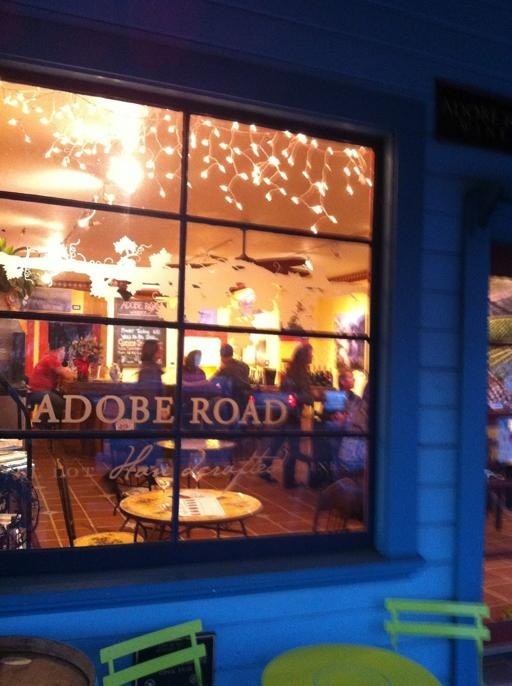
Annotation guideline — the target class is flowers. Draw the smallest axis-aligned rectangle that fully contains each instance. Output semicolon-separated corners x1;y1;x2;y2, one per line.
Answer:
66;329;105;369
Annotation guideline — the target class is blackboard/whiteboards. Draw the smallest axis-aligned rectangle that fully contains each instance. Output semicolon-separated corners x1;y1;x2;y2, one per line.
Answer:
114;297;166;368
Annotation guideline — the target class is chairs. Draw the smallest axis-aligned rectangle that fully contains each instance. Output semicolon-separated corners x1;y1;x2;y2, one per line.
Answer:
55;458;144;547
113;438;167;517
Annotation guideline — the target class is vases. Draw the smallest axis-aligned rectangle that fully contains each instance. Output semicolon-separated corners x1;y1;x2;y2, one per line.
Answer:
73;358;92;382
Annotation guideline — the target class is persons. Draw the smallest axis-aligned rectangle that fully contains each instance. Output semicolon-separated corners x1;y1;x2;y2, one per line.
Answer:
323;369;362;420
180;349;210;389
27;343;75;422
256;344;316;488
209;344;252;397
101;339;163;493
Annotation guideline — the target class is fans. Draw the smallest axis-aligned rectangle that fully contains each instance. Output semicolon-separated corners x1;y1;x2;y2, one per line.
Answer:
167;226;311;278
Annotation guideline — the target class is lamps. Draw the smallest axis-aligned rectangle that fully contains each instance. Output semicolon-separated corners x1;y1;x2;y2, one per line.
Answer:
117;288;133;302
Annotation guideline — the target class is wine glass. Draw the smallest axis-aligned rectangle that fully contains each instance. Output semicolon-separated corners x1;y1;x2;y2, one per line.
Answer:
189;453;207;495
154;458;173;507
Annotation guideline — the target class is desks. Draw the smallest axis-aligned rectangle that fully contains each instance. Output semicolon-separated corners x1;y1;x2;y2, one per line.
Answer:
117;488;263;543
154;437;239;489
